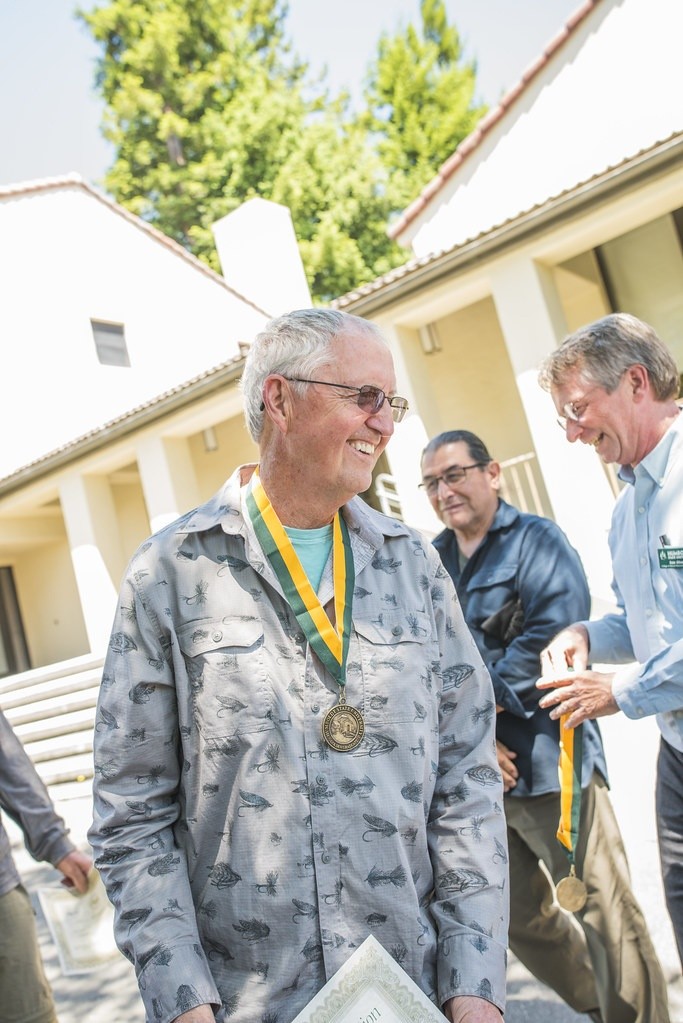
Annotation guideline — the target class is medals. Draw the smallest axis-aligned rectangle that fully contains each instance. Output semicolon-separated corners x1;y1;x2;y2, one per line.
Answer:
323;704;366;752
556;876;588;912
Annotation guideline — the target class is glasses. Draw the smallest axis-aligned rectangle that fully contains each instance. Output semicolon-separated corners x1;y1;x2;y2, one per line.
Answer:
419;462;486;492
260;378;409;423
556;371;626;431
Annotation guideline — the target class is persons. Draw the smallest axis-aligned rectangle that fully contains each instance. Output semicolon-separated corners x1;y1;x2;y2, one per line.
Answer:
421;431;676;1022
91;312;512;1023
0;705;94;1023
535;312;683;991
